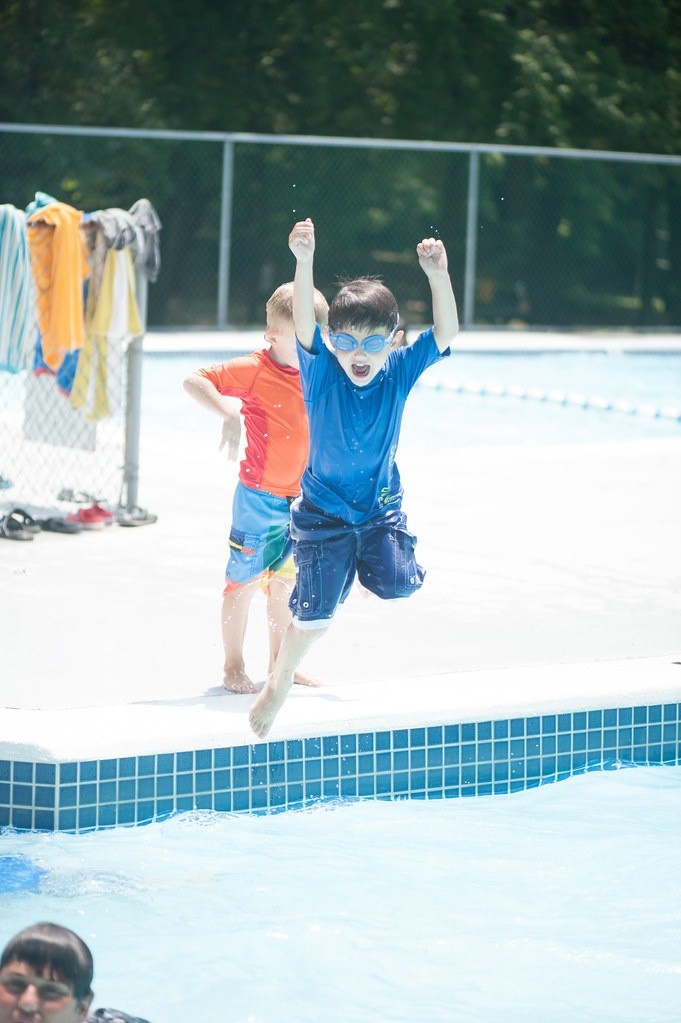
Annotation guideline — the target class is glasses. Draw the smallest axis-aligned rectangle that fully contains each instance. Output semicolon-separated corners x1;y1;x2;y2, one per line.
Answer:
0;973;74;1001
327;324;398;353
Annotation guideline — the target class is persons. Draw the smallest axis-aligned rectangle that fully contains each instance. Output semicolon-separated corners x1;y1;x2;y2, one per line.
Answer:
249;216;462;739
0;922;152;1023
180;280;330;695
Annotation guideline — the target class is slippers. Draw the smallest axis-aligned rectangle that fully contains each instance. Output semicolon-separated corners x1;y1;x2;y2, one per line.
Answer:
89;504;114;526
8;509;42;533
117;510;158;526
116;504;148;518
67;508;106;530
35;519;80;534
0;515;34;541
